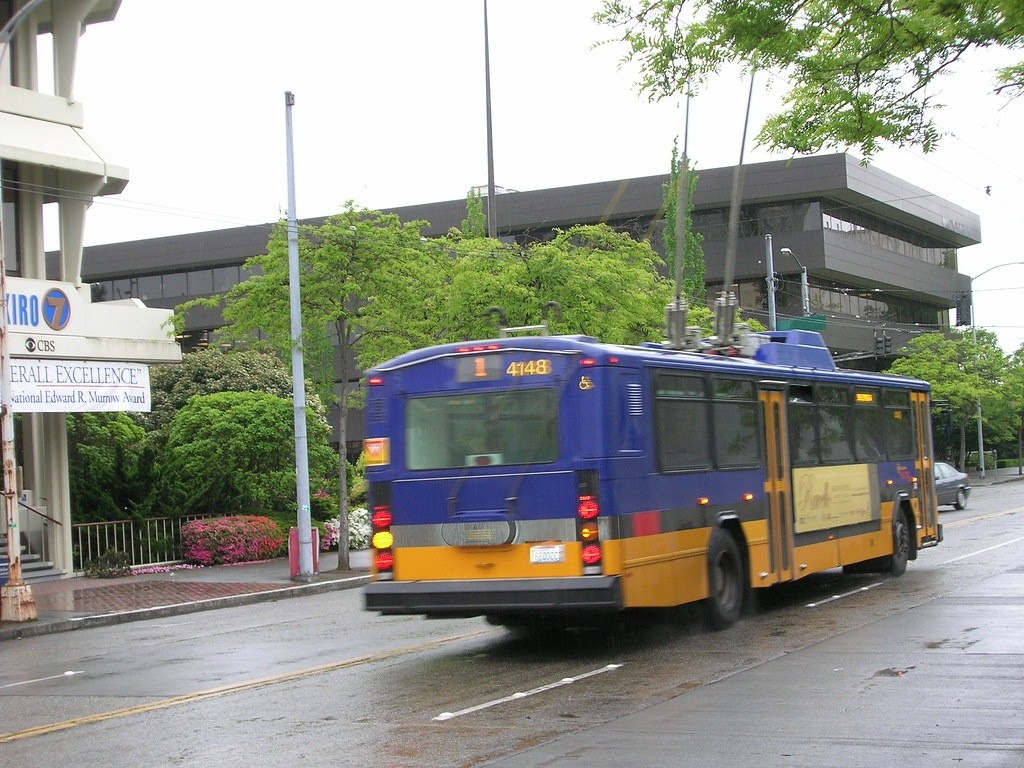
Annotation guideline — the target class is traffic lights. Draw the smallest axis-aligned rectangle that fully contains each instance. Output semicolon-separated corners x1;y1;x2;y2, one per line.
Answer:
883;331;892;357
874;332;882;357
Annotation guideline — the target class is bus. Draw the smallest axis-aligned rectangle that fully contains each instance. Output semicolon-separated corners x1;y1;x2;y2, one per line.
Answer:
363;329;939;631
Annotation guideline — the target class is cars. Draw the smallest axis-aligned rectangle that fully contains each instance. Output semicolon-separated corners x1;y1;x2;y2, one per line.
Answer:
933;461;969;510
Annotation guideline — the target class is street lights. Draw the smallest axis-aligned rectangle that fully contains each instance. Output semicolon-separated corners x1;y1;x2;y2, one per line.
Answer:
781;247;811;318
